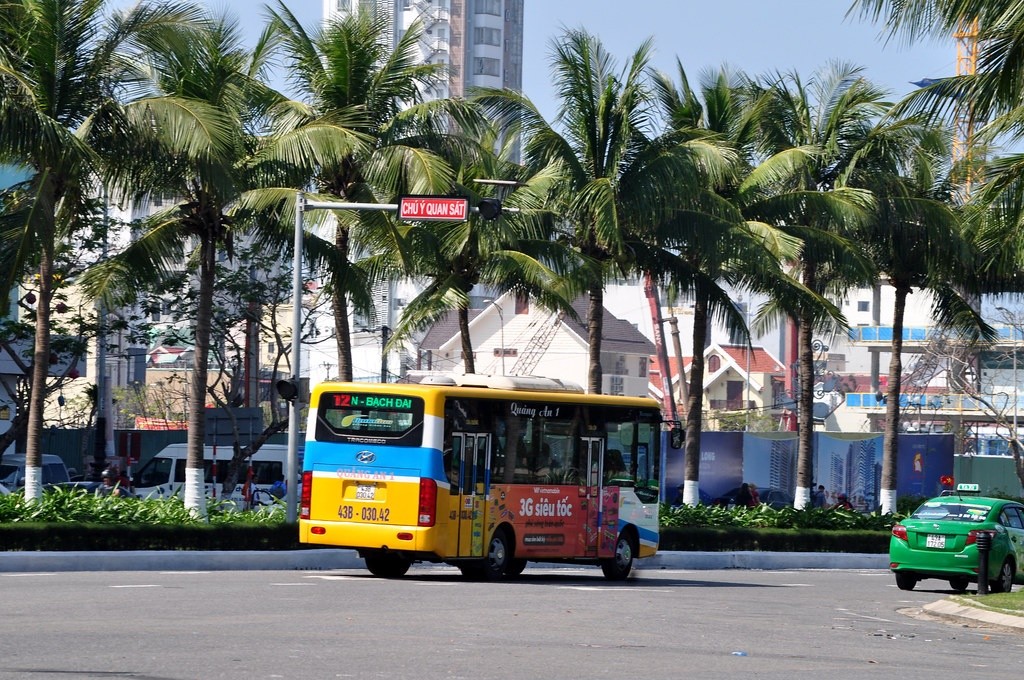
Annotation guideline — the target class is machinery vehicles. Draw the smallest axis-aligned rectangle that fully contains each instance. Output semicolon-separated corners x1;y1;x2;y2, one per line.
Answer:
644;273;689;429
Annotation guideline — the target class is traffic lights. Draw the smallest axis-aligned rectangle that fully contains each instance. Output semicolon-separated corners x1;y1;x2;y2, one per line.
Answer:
276;379;297;400
477;197;501;219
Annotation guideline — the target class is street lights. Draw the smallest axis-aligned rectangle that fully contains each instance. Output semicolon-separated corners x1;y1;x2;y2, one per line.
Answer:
994;305;1018;458
484;300;505;376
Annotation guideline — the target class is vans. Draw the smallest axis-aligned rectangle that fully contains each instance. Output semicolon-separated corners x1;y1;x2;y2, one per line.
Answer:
131;442;288;512
0;454;69;492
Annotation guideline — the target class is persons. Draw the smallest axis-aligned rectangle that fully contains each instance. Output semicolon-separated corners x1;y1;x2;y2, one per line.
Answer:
813;485;827;507
835;494;853;509
465;439;560;476
737;483;760;508
268;473;287;505
602;448;627;481
251;475;260;509
64;463;131;486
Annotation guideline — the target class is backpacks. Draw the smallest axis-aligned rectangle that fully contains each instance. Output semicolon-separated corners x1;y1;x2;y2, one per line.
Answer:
813;490;823;502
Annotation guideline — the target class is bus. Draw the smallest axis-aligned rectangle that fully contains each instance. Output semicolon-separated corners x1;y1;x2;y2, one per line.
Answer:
299;373;683;583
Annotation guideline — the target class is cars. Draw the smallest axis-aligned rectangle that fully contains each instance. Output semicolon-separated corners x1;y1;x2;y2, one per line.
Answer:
889;483;1024;593
44;481;136;499
